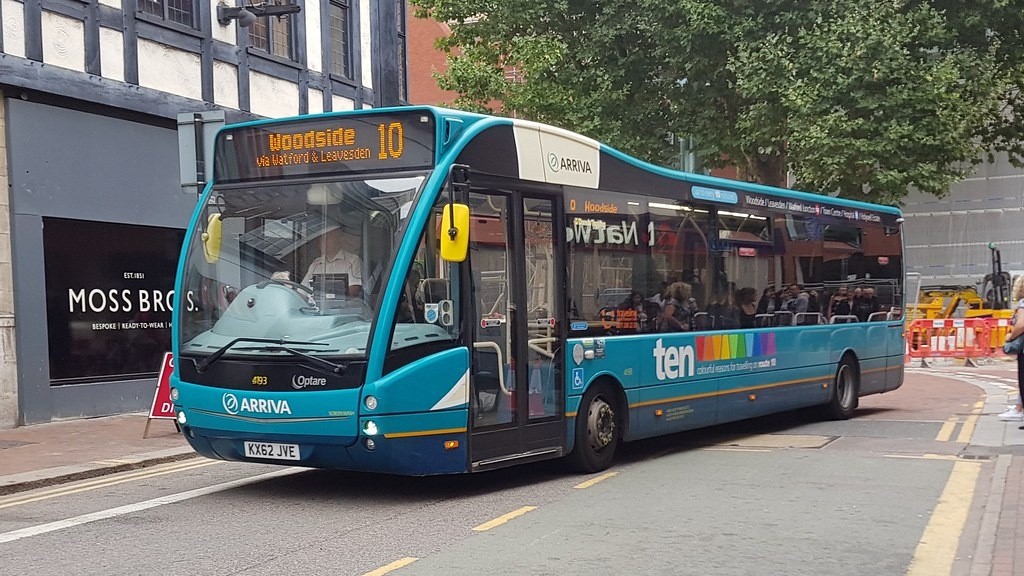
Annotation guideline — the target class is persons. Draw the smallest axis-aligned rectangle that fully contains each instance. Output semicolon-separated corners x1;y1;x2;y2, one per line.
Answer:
996;272;1024;432
610;282;878;333
297;224;365;302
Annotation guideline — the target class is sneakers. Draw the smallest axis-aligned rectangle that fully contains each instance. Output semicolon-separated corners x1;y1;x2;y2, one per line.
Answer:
1006;405;1017;411
997;409;1023;421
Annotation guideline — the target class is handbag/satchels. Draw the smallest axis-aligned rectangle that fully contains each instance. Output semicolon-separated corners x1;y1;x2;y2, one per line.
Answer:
1003;331;1024;354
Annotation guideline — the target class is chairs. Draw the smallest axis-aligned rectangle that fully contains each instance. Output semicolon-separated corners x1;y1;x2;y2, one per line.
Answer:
371;259;900;338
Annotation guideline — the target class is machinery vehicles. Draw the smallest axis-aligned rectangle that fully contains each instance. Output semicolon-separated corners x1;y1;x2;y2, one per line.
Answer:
917;245;1017;319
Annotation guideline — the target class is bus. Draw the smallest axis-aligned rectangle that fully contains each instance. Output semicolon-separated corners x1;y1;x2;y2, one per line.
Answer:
166;103;909;472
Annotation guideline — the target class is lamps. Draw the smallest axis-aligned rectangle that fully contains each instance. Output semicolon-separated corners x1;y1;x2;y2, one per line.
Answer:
217;1;257;27
